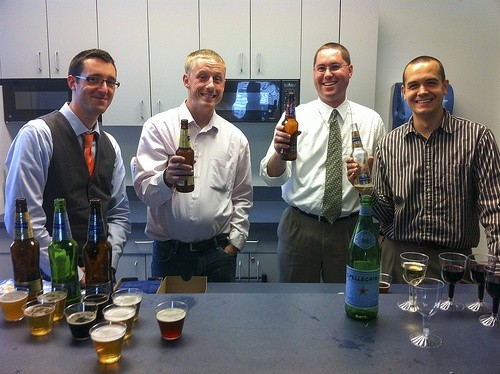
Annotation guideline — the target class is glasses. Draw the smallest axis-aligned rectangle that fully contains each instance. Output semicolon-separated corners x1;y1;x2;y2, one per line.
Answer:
75;76;120;89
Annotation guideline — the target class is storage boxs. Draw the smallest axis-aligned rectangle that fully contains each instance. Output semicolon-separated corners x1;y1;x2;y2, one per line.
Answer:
156;276;208;294
112;277;163;292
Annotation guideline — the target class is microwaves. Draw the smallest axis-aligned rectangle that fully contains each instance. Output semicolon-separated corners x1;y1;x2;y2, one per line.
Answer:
215;78;300;123
3;78;73;123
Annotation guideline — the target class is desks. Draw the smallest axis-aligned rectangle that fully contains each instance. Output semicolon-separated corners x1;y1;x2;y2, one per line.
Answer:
0;283;500;374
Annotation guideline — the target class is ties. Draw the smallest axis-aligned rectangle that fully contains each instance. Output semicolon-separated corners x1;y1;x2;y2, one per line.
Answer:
323;111;342;224
82;132;94;176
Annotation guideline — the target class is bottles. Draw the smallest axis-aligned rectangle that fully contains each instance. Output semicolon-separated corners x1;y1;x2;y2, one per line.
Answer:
281;92;299;161
349;122;373;191
81;199;113;315
48;199;82;307
346;195;381;321
9;198;44;306
175;119;194;193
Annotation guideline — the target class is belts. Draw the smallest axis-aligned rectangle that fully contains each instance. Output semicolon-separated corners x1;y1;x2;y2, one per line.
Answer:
159;237;227;253
295;207;358;223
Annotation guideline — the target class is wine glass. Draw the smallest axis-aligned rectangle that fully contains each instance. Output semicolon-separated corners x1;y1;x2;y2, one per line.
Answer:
479;264;500;328
463;254;497;314
410;278;443;348
436;253;468;311
399;251;429;313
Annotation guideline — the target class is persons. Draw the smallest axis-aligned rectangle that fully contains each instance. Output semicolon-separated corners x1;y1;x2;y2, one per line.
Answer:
135;48;253;283
4;49;131;287
260;43;386;281
345;56;500;284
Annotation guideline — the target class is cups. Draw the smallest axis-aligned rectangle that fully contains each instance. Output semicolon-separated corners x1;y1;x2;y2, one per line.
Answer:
81;288;111;321
154;300;188;340
89;320;127;365
21;299;56;336
0;288;29;321
36;287;69;321
112;288;143;320
378;273;392;293
103;304;137;341
63;302;98;341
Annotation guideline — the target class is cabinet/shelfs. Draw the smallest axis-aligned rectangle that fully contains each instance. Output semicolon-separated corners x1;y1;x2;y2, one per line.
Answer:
199;0;300;79
0;0;98;80
299;0;341;104
96;0;200;127
115;224;153;282
235;231;280;283
